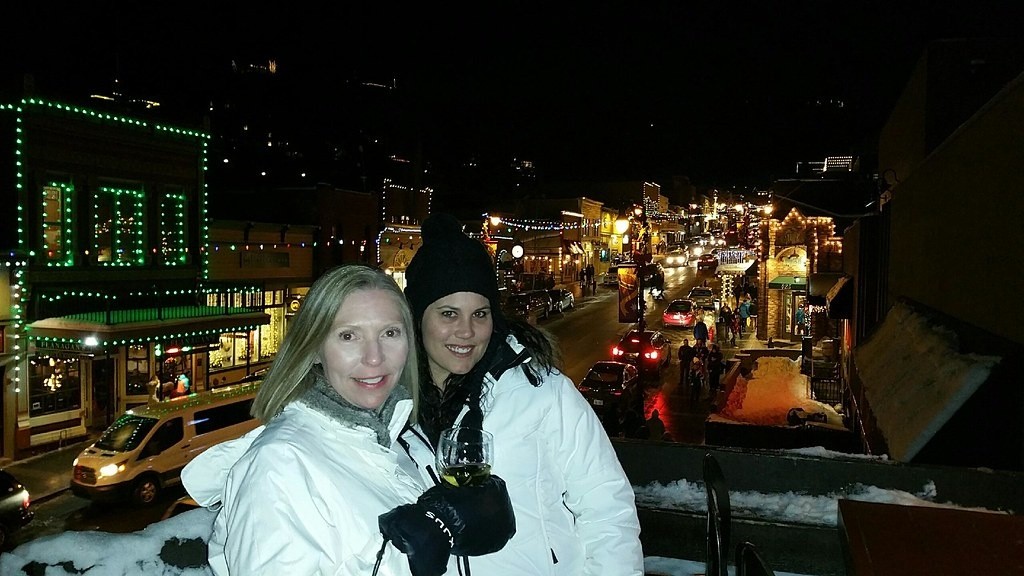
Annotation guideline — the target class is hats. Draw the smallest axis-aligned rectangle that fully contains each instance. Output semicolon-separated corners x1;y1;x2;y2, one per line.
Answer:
405;211;503;320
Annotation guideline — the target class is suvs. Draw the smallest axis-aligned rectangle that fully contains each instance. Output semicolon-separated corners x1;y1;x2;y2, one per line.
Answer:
501;289;553;323
613;329;671;382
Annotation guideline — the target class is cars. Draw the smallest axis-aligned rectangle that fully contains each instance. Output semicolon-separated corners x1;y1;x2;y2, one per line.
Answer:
604;266;618;287
662;299;699;330
0;469;35;549
546;289;575;313
709;247;729;262
698;254;718;270
687;286;714;312
578;360;638;414
667;229;741;253
644;262;664;286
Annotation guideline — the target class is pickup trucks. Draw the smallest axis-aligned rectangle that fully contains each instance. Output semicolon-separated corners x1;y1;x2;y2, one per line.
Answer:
667;250;690;267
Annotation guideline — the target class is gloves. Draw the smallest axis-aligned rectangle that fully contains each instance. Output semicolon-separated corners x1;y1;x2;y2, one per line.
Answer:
370;466;518;576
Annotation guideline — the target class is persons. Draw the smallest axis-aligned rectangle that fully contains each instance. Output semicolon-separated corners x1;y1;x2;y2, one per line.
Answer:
678;315;724;414
534;267;556;290
207;264;517;576
796;305;806;334
579;263;595;289
180;239;646;576
645;409;665;436
718;282;758;348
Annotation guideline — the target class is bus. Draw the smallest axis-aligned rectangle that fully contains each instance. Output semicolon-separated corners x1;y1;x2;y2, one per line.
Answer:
70;379;264;508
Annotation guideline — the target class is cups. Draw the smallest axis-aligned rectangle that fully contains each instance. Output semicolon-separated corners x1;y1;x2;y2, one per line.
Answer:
436;426;493;486
